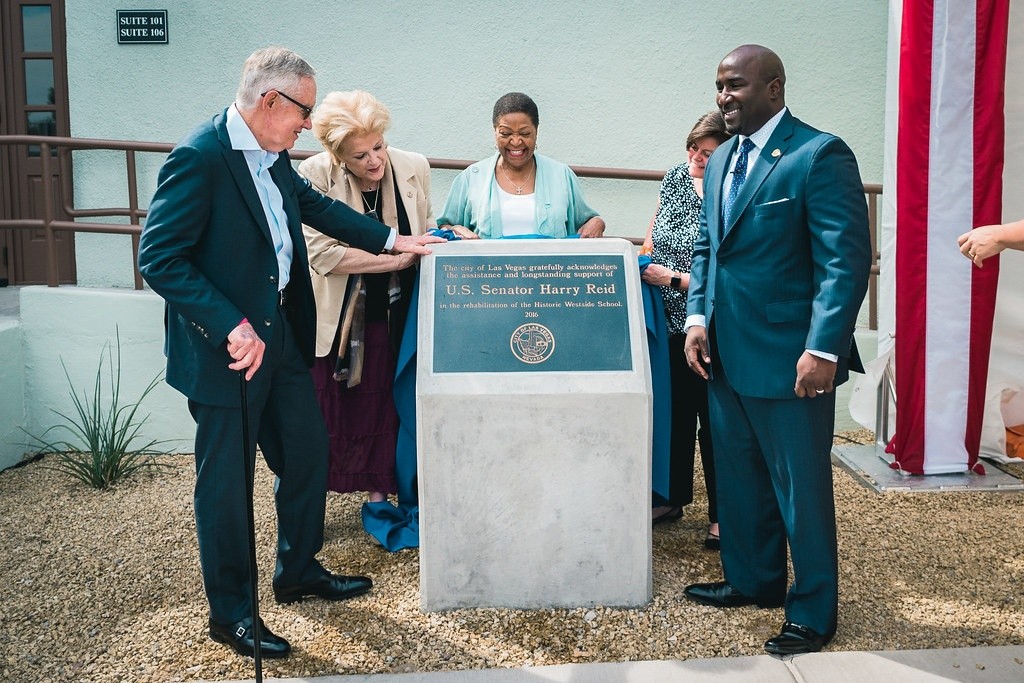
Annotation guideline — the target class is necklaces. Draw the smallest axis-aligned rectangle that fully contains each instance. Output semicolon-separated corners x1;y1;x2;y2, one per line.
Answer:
358;177;374;191
500;157;534;195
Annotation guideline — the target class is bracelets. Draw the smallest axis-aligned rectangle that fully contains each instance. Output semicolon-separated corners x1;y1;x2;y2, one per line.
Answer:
451;224;461;231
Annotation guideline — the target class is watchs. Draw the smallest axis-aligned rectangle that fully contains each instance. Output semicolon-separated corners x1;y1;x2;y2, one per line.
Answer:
669;272;682;290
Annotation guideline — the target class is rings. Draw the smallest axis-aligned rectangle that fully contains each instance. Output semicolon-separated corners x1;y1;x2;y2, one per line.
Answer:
968;250;975;258
816;389;824;394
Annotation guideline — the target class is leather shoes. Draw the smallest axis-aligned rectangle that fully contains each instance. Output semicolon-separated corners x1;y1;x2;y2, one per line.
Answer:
209;615;292;660
764;620;824;654
684;581;783;610
273;571;372;603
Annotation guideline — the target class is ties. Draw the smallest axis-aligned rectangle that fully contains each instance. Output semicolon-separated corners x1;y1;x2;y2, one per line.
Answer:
721;138;756;237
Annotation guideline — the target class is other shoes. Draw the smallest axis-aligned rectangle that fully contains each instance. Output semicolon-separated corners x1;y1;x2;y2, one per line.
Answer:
652;506;683;525
704;530;720;550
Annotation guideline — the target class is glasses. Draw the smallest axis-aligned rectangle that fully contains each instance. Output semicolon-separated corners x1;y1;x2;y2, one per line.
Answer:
262;89;312;120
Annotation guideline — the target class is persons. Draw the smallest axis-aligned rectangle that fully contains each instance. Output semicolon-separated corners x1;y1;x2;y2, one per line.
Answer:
296;90;439;545
136;48;449;661
681;44;873;657
958;218;1024;268
643;110;735;551
436;93;605;239
